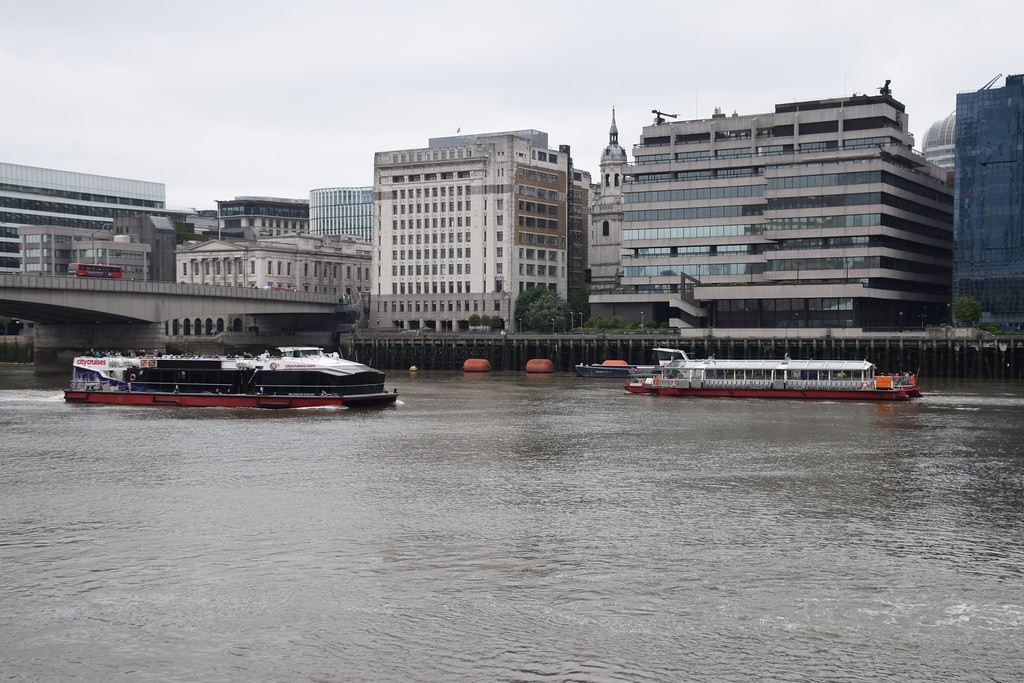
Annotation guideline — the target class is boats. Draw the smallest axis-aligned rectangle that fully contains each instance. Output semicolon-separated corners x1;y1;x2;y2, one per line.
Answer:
64;347;400;409
575;347;923;401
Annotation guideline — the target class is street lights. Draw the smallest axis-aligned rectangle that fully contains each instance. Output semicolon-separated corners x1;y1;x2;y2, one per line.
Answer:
579;312;582;327
519;319;521;335
571;312;573;329
947;304;951;327
552;319;554;334
917;314;927;331
900;312;903;333
795;312;799;327
641;312;643;331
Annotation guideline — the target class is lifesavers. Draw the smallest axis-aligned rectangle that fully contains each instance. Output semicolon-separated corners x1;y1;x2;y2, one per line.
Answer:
269;362;277;369
129;373;137;381
860;381;870;389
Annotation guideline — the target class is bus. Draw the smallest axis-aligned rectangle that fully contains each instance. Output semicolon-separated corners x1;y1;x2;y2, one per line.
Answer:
68;262;123;279
